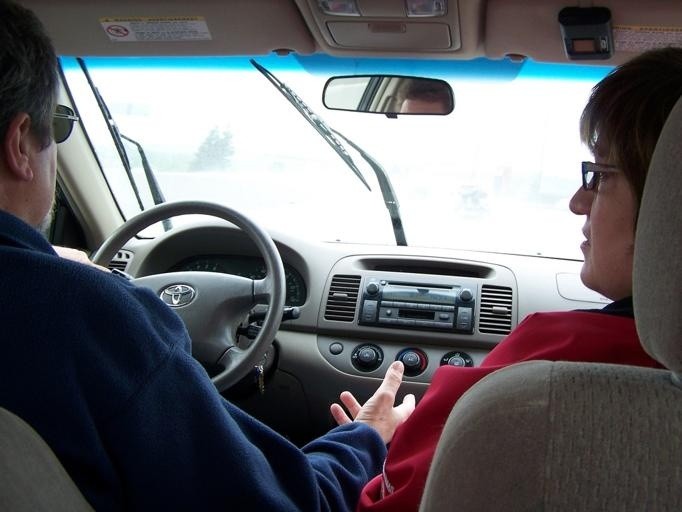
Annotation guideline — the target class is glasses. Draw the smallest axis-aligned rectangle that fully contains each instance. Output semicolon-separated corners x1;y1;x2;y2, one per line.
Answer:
49;104;79;144
580;161;625;190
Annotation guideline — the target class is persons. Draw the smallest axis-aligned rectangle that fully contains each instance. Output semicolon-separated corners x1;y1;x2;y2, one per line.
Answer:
354;47;682;512
0;2;419;512
399;78;452;113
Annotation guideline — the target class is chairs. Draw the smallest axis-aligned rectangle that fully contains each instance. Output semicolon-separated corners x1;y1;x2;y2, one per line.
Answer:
0;401;88;508
418;94;682;508
